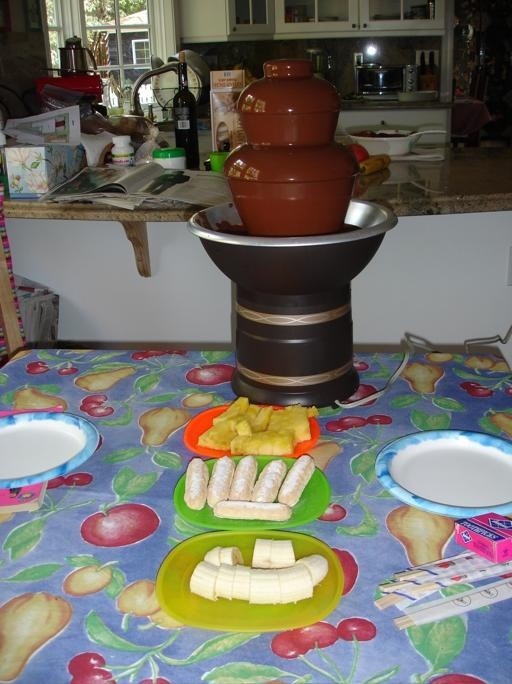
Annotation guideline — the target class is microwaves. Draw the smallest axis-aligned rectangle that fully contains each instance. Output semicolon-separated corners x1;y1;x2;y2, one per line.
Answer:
353;64;417;101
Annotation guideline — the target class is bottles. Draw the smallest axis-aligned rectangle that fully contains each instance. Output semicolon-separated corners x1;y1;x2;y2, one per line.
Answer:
111;135;134;169
152;51;200;171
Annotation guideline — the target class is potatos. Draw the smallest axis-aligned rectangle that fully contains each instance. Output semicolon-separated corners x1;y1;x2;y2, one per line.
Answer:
360;153;391;175
358;168;390;188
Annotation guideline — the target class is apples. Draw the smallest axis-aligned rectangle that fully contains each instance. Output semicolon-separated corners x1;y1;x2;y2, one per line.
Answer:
345;144;369;166
352;175;368;197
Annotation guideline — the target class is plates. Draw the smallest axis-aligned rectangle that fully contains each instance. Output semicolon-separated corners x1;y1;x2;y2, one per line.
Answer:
182;403;322;458
1;412;99;485
173;454;332;530
374;428;512;522
156;528;344;636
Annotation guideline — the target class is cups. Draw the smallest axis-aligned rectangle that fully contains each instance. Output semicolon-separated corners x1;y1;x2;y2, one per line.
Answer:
210;152;229;173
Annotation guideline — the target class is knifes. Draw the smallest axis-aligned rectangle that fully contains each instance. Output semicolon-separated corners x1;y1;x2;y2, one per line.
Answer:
420;50;435;75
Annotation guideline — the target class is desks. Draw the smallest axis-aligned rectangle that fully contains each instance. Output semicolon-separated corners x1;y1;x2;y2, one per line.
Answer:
0;346;512;684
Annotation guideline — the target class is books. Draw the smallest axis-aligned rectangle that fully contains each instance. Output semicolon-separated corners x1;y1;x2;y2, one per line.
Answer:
39;162;230;212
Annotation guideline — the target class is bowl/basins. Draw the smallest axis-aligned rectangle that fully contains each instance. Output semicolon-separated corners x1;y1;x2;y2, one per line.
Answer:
397;90;439;104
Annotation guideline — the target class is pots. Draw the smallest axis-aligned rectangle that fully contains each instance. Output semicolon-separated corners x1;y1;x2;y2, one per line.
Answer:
348;129;448;157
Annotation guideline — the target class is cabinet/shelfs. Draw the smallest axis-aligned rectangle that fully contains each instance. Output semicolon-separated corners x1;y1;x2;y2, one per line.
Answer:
157;129;212;152
148;0;452;108
335;108;454;144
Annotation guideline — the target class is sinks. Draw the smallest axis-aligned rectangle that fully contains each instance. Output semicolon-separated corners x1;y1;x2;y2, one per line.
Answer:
154;124;213;172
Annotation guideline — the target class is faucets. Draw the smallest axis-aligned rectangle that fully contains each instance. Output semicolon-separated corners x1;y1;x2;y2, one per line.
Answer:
130;62;178;117
